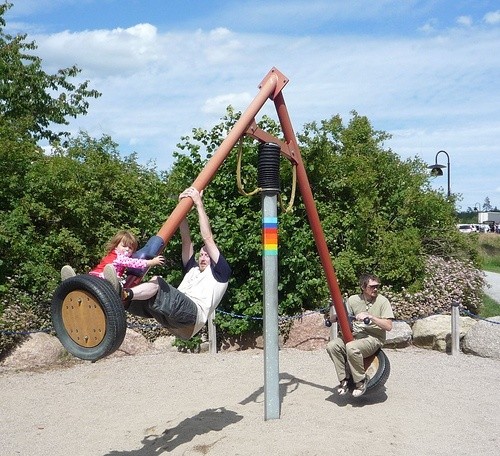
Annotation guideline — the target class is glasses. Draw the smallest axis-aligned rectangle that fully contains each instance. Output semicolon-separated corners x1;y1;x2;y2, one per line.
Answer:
366;284;380;290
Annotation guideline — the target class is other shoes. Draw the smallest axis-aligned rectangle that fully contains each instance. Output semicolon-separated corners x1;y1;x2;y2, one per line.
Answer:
352;375;370;398
60;265;75;281
337;374;352;396
103;263;133;310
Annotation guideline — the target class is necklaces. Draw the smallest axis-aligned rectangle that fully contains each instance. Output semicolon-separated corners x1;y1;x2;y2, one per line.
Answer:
362;292;373;310
89;230;165;279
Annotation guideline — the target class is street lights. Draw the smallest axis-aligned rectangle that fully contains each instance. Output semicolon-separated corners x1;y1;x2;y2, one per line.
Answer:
427;150;451;203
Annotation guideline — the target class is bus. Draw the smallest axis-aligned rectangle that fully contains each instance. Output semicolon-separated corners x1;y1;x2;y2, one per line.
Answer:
483;221;495;232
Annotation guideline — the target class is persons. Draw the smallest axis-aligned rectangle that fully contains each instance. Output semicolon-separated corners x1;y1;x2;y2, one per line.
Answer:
60;186;233;342
326;273;396;396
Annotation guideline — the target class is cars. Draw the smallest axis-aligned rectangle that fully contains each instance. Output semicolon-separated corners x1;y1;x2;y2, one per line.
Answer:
495;225;500;233
454;224;491;234
